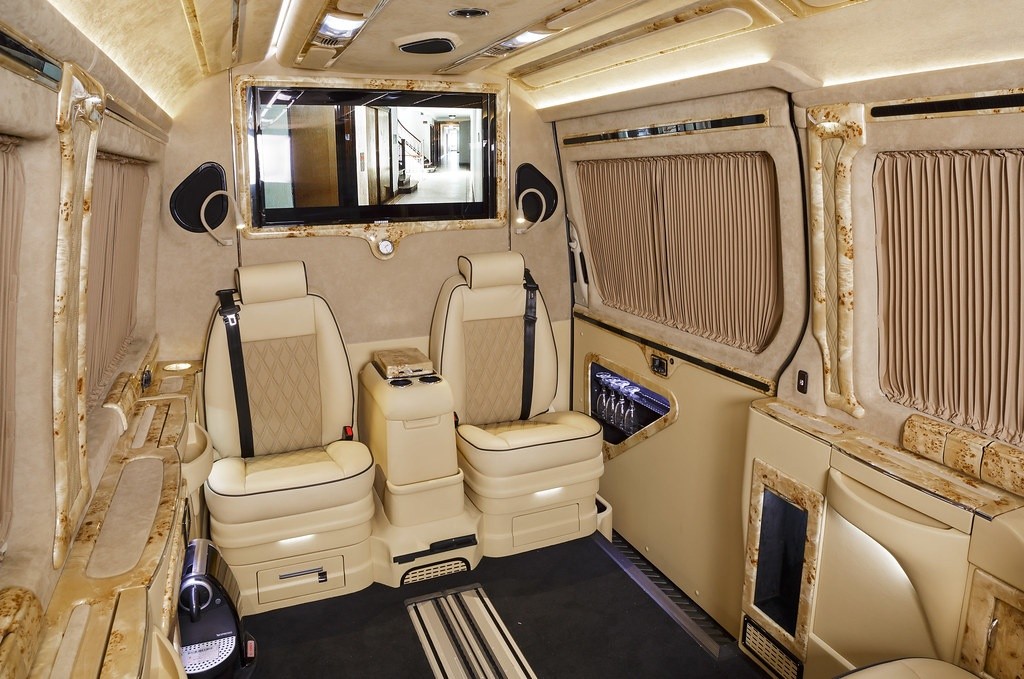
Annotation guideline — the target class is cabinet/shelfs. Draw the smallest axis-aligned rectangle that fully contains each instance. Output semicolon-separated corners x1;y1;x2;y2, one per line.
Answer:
590;362;670;444
802;439;986;678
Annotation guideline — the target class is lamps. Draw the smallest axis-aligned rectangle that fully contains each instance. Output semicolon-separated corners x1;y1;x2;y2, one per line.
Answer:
515;188;546;234
199;190;245;246
318;13;367;39
499;31;554;48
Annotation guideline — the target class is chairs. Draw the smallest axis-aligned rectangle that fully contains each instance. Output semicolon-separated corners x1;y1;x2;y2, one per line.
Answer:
202;260;375;549
832;657;983;679
429;251;605;499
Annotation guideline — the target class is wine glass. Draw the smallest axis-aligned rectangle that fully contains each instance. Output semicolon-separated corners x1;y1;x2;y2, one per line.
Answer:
596;372;611;417
604;377;620;425
614;381;630;428
623;386;640;437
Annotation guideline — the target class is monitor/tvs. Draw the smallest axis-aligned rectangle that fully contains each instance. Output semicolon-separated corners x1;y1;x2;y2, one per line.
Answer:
251;85;497;227
